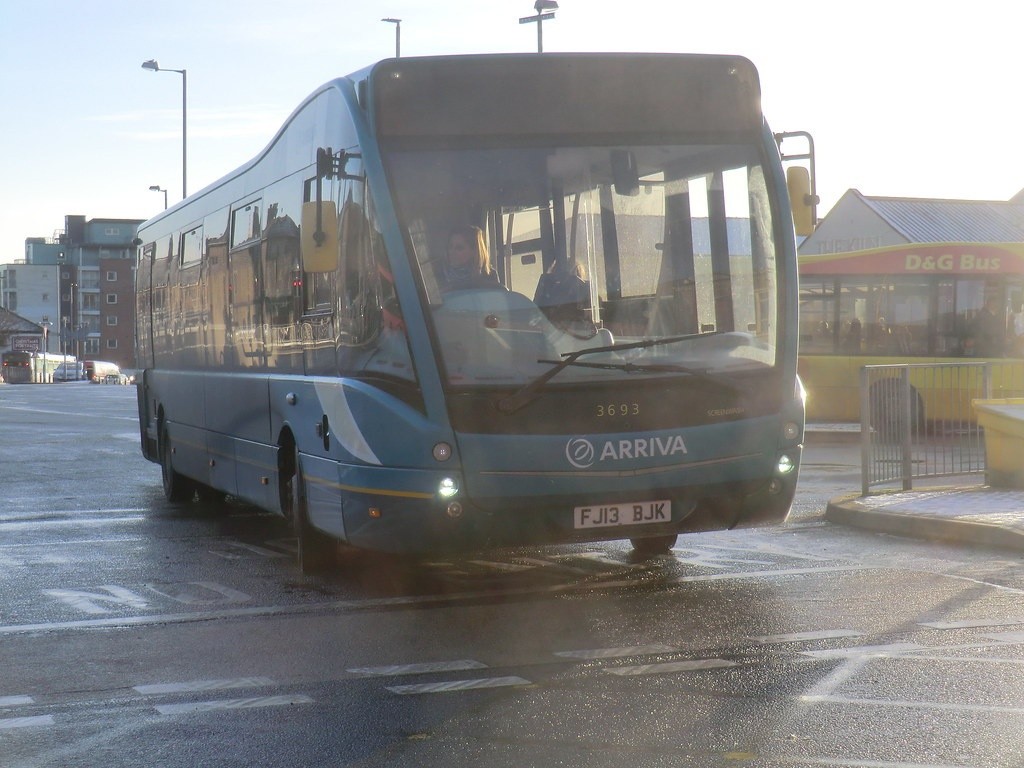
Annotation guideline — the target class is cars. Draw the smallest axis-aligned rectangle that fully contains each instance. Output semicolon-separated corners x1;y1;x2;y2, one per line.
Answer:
52;363;83;385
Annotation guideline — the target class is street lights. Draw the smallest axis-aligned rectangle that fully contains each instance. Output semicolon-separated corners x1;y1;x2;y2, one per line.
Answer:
379;18;400;58
149;186;167;210
142;60;188;200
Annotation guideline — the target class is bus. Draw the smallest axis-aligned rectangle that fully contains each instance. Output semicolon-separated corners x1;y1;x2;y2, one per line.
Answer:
791;240;1024;434
82;360;120;380
133;53;819;574
2;350;78;384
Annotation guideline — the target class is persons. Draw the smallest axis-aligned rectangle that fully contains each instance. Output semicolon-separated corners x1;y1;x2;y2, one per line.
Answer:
431;224;597;298
